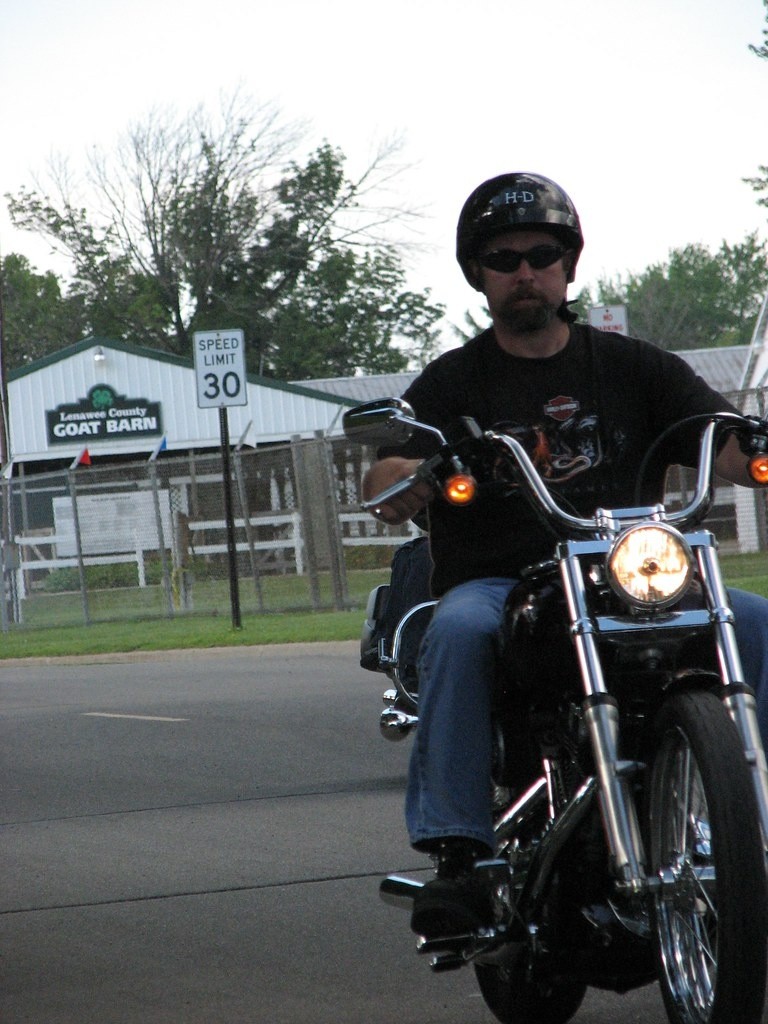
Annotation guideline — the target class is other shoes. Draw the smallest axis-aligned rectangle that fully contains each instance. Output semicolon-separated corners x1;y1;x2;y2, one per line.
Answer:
411;837;492;936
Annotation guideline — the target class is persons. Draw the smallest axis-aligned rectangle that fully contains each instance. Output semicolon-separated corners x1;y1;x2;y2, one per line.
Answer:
360;173;768;944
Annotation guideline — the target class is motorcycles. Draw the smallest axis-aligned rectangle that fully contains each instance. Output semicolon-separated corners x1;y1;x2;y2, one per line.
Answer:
324;401;768;1024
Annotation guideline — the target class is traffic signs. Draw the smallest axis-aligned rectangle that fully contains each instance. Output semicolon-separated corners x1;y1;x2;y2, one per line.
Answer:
192;329;248;409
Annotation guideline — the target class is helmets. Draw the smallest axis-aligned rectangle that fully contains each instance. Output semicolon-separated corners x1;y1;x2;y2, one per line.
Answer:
456;172;584;262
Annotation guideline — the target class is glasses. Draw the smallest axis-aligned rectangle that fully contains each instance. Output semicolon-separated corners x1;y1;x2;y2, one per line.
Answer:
478;245;568;273
